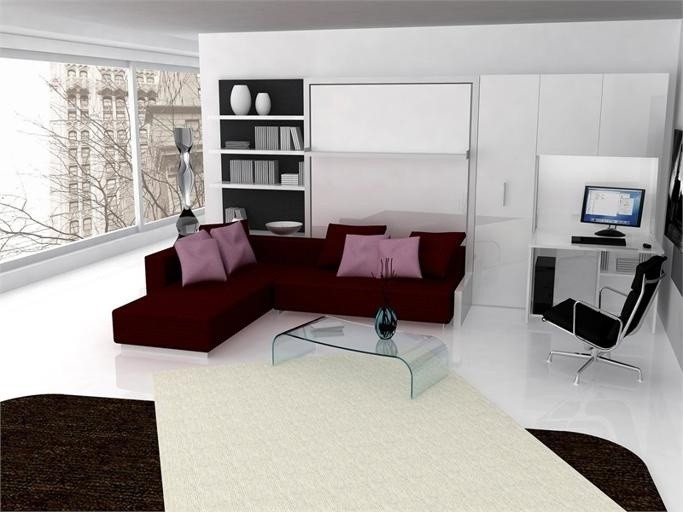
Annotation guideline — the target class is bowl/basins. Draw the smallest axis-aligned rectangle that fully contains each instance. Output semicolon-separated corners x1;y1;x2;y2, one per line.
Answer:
266;221;302;236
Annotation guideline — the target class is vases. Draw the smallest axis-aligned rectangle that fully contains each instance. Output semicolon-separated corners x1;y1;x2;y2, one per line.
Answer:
229;83;272;116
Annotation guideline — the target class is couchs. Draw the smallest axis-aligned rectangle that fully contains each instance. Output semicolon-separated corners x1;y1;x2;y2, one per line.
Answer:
112;238;473;360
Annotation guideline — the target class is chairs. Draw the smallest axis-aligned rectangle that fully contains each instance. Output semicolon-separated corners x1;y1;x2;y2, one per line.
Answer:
541;255;667;385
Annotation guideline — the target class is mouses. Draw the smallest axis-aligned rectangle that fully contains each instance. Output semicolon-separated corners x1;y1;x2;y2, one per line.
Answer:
642;243;651;249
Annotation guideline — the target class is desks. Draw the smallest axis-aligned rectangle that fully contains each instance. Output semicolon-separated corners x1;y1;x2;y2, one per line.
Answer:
528;226;664;334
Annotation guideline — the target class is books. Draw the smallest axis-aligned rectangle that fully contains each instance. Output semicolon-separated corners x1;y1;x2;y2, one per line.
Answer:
225;206;251;231
223;140;250;149
229;158;304;186
253;126;303;151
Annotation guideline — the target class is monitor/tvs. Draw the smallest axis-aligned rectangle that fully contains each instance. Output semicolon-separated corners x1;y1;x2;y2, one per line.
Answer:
580;185;645;237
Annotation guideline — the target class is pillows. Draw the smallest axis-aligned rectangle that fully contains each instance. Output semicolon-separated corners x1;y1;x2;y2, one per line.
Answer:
335;234;422;280
172;219;258;286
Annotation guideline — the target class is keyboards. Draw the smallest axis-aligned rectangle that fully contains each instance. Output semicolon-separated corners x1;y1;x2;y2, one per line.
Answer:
571;235;627;247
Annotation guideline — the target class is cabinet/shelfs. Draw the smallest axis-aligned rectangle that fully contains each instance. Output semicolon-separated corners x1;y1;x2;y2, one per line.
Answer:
472;20;683;313
199;25;310;236
